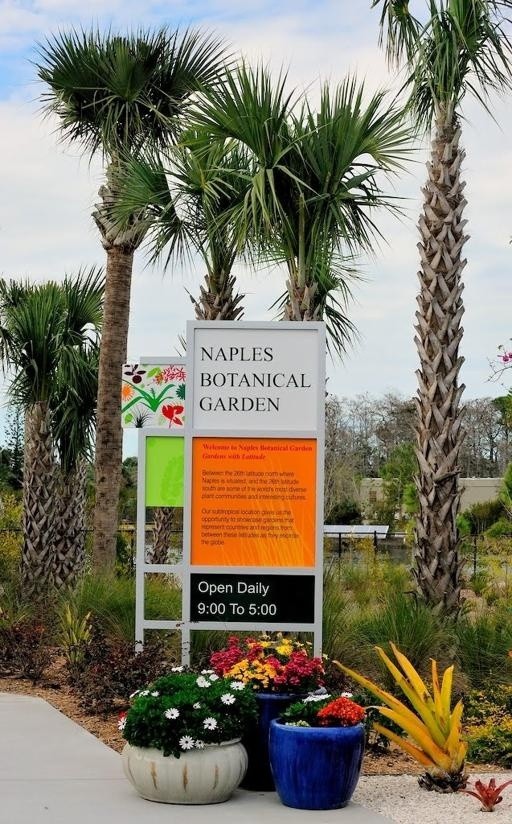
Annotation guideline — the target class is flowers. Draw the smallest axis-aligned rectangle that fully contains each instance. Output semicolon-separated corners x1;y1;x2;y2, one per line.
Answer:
117;665;260;759
210;632;332;692
280;690;365;727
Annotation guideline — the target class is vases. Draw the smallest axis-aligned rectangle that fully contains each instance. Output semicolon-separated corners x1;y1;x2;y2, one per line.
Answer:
269;717;367;810
240;692;308;792
122;737;248;805
